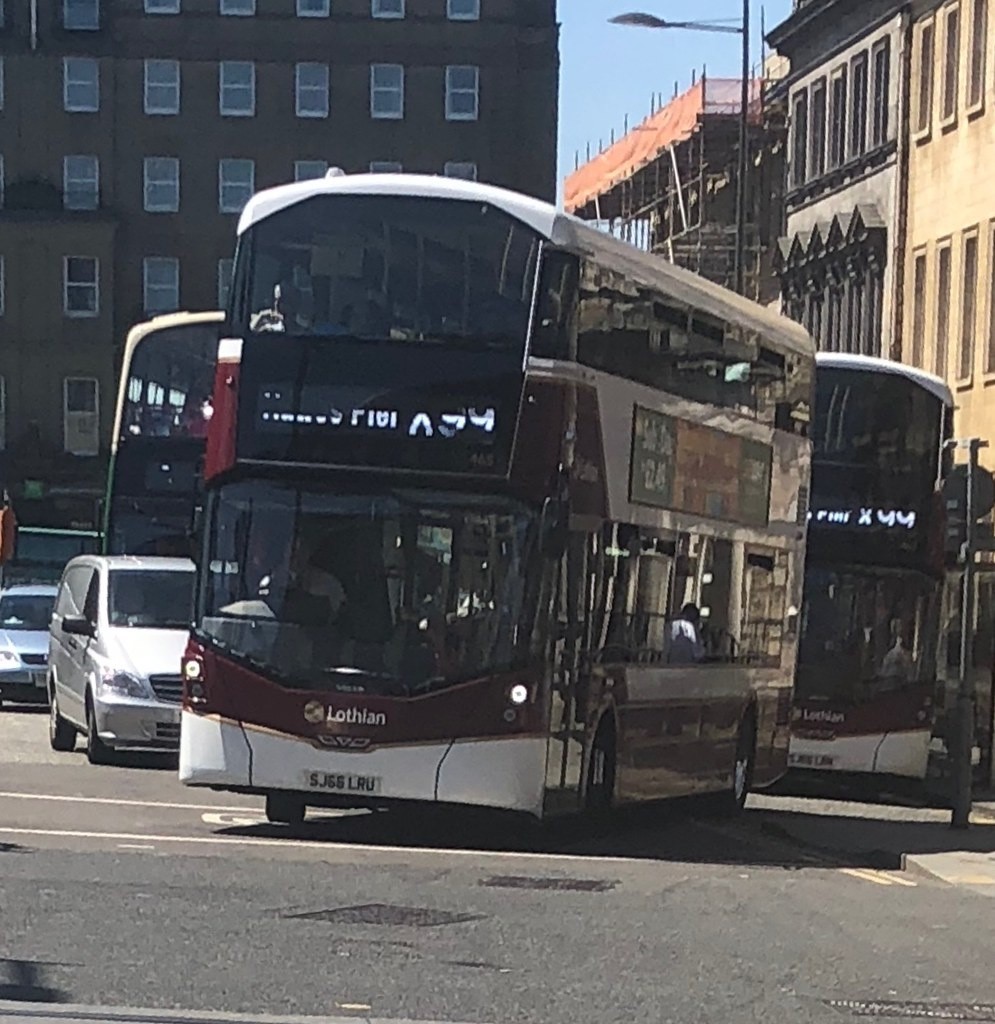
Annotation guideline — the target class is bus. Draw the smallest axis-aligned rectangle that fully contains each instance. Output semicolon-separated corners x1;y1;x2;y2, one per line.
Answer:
175;163;819;850
100;306;225;556
789;350;960;790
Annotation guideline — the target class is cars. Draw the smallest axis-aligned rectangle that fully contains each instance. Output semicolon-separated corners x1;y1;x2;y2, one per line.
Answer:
1;585;59;709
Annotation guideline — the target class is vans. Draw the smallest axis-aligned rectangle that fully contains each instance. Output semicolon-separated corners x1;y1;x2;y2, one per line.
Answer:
45;553;194;767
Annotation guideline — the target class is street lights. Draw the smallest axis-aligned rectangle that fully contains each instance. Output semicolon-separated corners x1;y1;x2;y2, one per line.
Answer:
607;12;750;293
